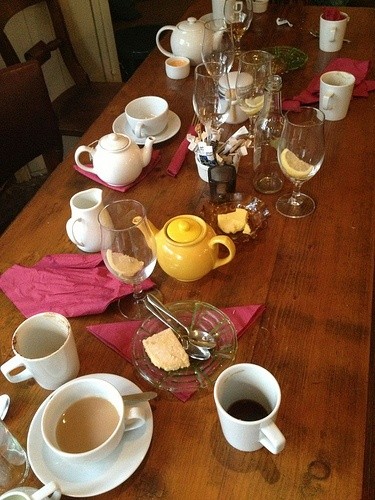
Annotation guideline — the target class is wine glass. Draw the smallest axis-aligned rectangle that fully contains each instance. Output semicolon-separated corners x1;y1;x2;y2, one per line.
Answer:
100;200;164;320
275;106;326;218
192;0;276;151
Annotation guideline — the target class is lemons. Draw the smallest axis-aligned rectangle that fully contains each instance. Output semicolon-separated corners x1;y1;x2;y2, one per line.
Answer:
106;249;144;276
241;95;264;113
280;149;313;177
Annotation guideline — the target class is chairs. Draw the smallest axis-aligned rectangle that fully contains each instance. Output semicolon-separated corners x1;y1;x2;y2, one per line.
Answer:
109;0;194;80
0;60;64;176
0;0;125;138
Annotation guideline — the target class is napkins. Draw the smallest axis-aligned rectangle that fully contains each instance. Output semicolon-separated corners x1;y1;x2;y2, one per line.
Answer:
0;252;155;320
166;125;201;177
86;303;264;402
72;149;160;194
283;58;375;111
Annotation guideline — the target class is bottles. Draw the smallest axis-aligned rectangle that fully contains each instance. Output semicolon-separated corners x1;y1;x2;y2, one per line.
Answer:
253;76;284;194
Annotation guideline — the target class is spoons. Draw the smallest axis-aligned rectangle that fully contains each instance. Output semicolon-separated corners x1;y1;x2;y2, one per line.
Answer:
142;295;217;360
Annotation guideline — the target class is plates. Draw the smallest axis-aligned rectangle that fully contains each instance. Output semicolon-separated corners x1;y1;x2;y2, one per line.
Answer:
26;372;153;497
112;110;182;145
260;47;308;71
201;192;270;243
131;301;237;393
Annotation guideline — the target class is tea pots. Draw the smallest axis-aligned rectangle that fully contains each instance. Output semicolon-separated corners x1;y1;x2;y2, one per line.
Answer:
155;17;224;66
131;214;237;283
75;132;154;187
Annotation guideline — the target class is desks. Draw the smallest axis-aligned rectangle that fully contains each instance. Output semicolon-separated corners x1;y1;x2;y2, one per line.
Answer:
0;0;375;500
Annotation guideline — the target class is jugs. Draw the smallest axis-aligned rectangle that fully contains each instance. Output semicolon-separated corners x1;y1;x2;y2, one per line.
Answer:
65;188;114;253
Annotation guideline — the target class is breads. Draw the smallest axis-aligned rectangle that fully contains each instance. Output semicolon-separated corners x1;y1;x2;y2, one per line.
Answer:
143;328;190;371
217;208;254;237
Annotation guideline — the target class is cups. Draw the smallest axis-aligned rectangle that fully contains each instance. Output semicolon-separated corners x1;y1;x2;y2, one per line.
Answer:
214;362;285;453
125;95;169;139
318;12;348;52
316;71;355;121
0;310;80;392
165;56;191;79
217;71;254;125
212;0;243;29
209;166;237;203
195;141;240;182
41;379;147;462
0;481;62;500
